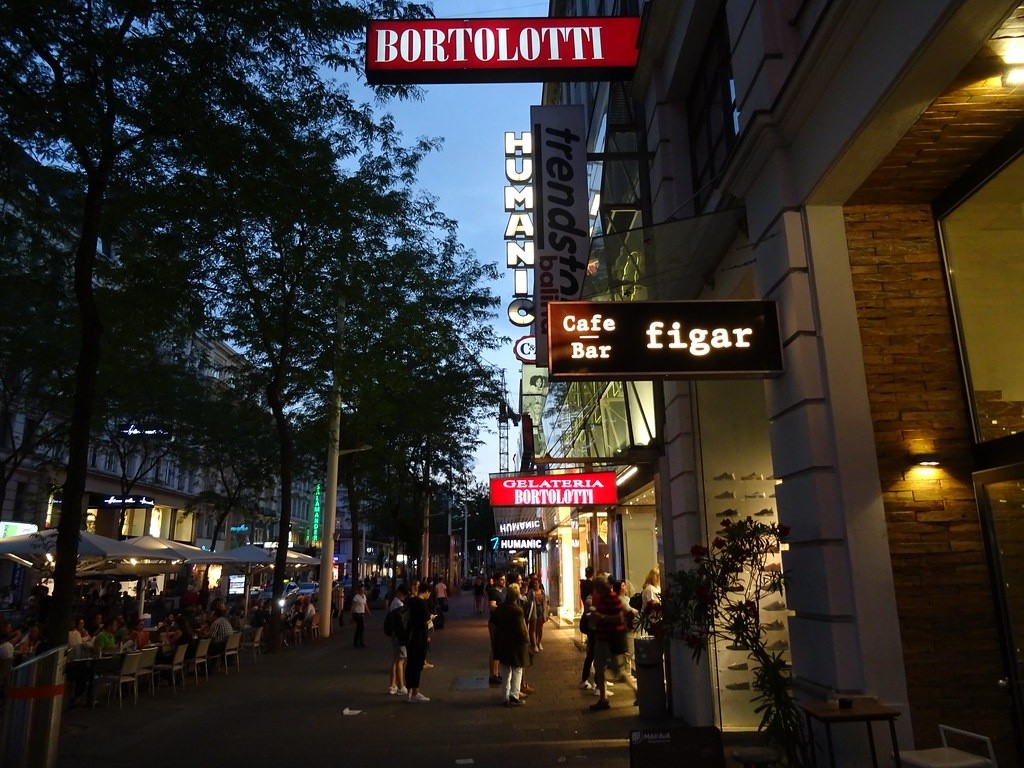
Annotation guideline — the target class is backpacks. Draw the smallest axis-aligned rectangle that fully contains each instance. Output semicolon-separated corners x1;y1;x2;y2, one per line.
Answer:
387;597;423;646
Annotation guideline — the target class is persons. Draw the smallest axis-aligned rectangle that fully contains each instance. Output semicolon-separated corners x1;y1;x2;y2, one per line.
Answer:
229;572;387;650
0;570;241;705
384;571;449;704
488;571;548;706
461;572;494;615
578;566;661;709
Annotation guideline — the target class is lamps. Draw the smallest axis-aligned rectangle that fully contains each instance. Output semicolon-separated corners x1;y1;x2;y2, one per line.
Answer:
913;453;944;466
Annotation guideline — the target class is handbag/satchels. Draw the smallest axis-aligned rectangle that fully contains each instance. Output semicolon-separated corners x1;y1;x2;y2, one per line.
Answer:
444;601;448;612
580;613;592;634
384;612;394;637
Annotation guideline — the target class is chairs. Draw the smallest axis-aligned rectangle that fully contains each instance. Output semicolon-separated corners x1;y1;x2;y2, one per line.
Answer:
239;626;263;664
149;643;189;696
304;615;320;640
280;618;302;647
210;631;242;676
183;639;210;685
104;646;158;709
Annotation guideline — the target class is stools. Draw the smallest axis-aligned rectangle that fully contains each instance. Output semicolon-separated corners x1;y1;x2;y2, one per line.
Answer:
732;747;784;768
890;724;998;768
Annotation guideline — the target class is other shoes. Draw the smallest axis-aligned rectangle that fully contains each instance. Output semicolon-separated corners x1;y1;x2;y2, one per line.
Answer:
388;685;398;695
398;687;408;696
594;688;613;696
521;684;535;693
580;678;594;689
533;643;544;653
520;691;528;699
489;673;503;684
423;663;434;669
590;700;611;710
605;681;615;686
406;692;431;703
505;695;527;706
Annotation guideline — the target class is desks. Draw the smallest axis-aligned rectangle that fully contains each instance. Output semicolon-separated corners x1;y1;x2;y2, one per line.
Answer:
65;655;124;710
797;697;902;768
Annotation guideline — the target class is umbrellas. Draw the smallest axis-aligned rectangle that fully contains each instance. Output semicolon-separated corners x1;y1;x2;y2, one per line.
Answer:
0;529;321;619
